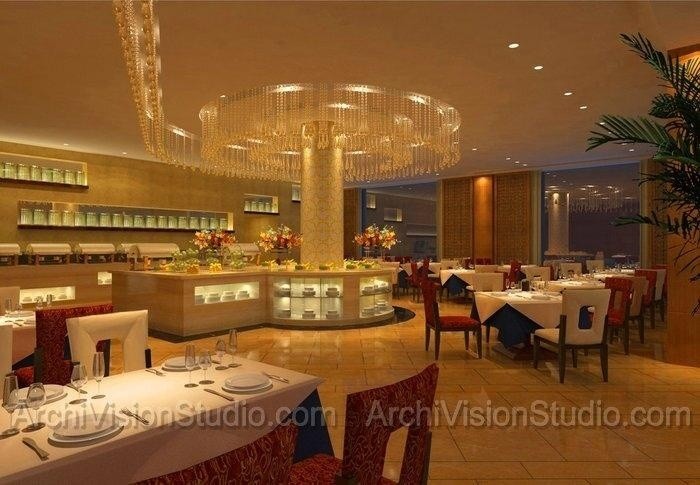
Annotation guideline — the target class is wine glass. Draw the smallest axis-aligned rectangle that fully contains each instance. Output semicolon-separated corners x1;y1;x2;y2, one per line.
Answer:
71;364;89;404
91;352;106;399
185;329;238;387
491;278;560;301
2;376;46;437
468;263;474;271
559;263;640;285
4;293;53;316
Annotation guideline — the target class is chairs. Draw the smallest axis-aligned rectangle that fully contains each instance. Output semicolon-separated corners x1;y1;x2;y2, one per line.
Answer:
290;361;439;485
0;286;21;315
65;309;149;381
13;303;114;389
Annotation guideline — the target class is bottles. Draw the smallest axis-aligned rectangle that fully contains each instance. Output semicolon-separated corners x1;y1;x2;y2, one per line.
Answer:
19;208;228;230
1;162;85;186
243;200;271;213
292;190;300;201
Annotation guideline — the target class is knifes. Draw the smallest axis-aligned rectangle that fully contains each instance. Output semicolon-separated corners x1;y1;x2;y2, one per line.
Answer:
67;383;87;394
204;389;233;401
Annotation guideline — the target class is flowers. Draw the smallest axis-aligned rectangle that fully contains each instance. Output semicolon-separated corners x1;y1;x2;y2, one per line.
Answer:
188;227;237;250
352;223;402;249
255;223;303;253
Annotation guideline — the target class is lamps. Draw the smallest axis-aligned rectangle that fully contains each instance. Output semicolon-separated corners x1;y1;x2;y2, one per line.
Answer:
110;0;462;183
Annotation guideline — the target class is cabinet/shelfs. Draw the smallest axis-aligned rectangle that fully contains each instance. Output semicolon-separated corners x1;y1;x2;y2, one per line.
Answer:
17;199;235;233
242;192;280;216
0;152;90;189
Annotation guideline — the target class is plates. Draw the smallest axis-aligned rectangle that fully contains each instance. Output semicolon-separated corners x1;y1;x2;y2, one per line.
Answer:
19;384;68;405
3;313;52;326
50;413;123;443
222;373;272;392
162;357;198;371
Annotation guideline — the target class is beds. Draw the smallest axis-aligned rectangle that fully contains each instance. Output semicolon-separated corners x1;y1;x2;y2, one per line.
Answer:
0;353;322;485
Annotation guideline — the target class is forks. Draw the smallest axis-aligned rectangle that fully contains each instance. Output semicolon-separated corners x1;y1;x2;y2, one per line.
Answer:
262;370;288;383
22;436;49;457
121;407;150;424
145;369;163;375
